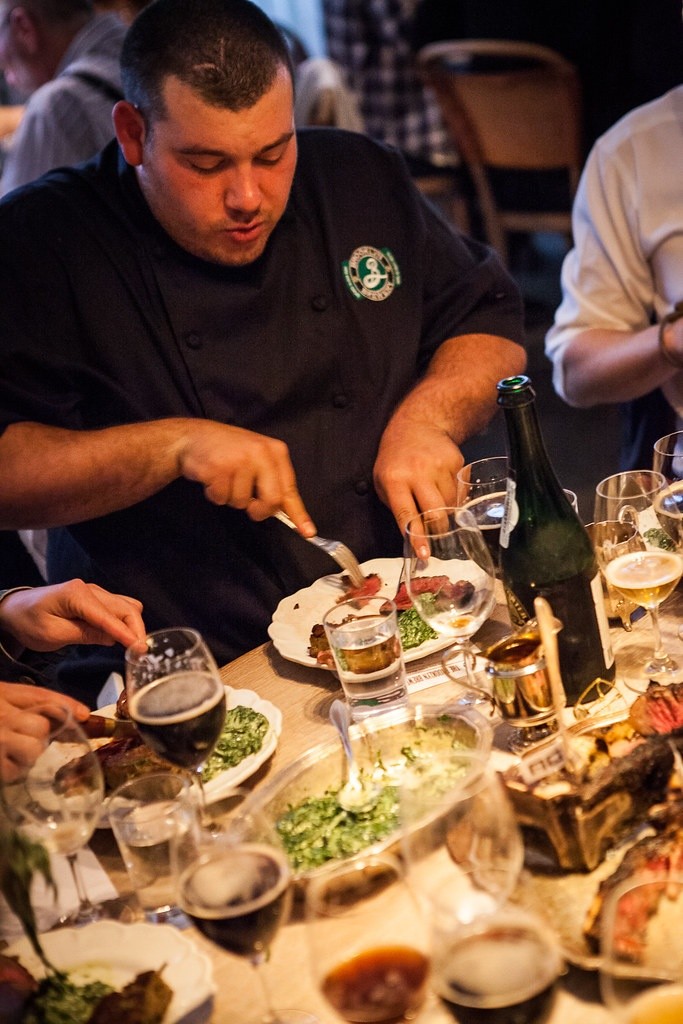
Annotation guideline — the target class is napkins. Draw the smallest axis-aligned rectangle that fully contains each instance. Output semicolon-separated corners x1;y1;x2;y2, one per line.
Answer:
0;846;121;939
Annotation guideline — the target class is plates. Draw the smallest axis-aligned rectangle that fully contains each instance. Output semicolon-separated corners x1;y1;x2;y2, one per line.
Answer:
267;556;495;671
29;686;281;829
15;922;215;1022
226;704;494;896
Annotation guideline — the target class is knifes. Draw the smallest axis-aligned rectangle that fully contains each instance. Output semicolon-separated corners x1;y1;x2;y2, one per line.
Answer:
390;544;414;605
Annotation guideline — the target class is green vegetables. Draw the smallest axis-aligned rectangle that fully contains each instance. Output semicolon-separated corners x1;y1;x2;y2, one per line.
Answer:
278;784;400;873
642;527;676;553
395;593;439;646
0;833;116;1024
199;705;269;782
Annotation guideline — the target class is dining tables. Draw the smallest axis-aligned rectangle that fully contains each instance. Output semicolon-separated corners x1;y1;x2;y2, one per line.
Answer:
0;572;683;1024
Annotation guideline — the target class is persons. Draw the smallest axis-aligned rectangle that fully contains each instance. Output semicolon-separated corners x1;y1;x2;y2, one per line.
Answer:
0;577;149;791
544;82;683;482
0;0;528;674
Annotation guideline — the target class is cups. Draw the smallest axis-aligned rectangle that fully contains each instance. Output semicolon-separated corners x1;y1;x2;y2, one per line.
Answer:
397;748;565;1022
104;770;210;930
0;429;683;1024
650;431;683;547
322;596;409;722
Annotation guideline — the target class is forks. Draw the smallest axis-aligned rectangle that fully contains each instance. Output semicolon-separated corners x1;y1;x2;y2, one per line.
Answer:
248;491;365;593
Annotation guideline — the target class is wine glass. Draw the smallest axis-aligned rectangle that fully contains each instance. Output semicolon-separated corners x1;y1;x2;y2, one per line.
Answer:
170;788;324;1024
404;505;505;725
123;625;246;842
593;470;683;692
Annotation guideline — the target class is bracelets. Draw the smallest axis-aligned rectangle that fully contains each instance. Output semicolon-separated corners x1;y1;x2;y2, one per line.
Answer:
657;300;683;368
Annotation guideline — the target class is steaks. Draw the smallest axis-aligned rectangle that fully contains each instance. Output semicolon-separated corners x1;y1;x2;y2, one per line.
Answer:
381;577;475;613
53;687;186;798
340;574;383;609
0;955;37;1024
88;971;173;1024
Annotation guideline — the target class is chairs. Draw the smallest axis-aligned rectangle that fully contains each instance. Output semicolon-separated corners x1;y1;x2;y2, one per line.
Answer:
308;40;582;258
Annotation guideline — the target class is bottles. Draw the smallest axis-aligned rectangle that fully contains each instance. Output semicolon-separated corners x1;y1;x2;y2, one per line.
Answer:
495;375;617;709
